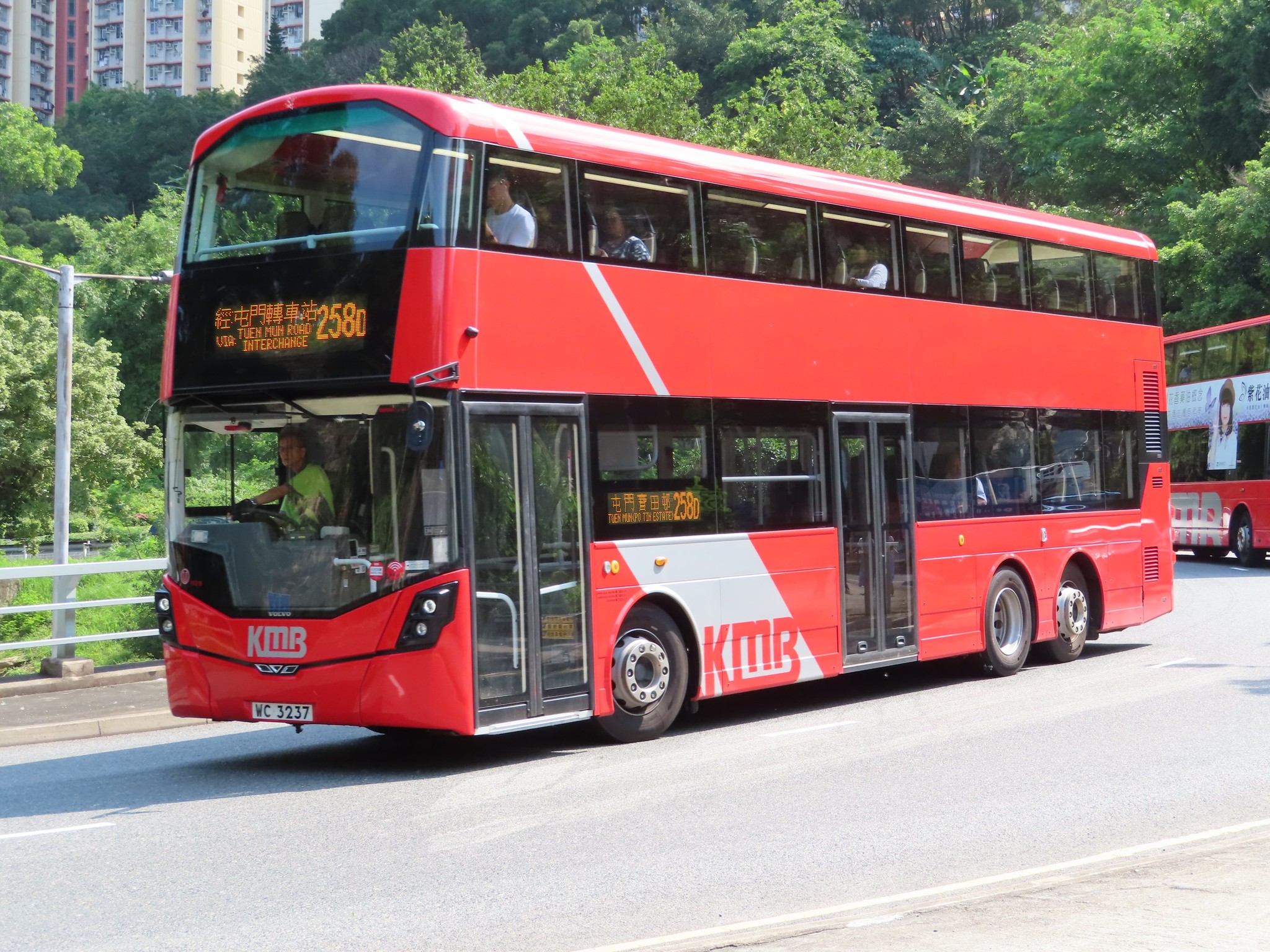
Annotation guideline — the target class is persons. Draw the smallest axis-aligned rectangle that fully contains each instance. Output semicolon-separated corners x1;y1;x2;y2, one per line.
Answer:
859;513;899;622
944;446;987;514
1207;379;1237;470
994;445;1091;507
1178;353;1198;383
483;173;558;247
227;425;335;540
846;241;887;289
594;204;667;261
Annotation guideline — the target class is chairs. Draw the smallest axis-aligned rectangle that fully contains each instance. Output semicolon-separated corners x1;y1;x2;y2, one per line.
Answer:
509;190;1133;316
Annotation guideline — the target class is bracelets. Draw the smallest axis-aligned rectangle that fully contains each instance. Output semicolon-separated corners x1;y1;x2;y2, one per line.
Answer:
599;249;604;257
485;234;495;242
849;277;855;287
250;498;256;505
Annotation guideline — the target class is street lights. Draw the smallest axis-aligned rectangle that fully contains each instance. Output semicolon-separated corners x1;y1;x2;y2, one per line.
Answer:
0;253;174;660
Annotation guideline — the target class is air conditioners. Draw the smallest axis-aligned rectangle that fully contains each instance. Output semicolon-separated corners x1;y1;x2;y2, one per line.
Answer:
155;0;174;71
206;0;212;72
104;3;117;78
36;0;45;119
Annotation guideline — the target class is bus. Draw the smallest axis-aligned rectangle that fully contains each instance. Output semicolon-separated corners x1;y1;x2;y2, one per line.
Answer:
148;85;1175;744
1162;315;1270;567
